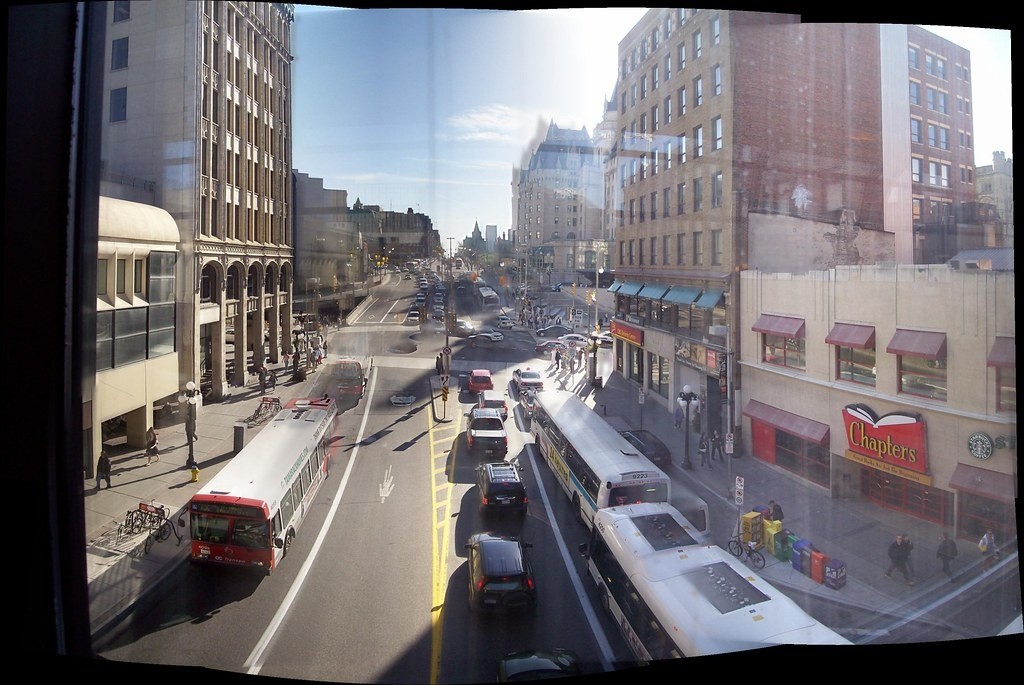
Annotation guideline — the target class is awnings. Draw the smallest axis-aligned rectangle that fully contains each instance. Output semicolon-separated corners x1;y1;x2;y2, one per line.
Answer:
743;399;830;446
885;329;947;360
607;279;725;310
825;324;876;351
949;463;1016;505
987;336;1016;369
751;315;805;338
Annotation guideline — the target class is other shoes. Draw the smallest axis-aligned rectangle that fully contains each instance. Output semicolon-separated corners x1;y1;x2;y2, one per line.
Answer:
906;581;914;584
883;573;892;578
146;463;150;466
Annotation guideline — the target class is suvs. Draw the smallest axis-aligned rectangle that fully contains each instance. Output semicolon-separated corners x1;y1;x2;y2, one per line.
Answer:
463;408;508;460
473;459;529;520
465;529;539;620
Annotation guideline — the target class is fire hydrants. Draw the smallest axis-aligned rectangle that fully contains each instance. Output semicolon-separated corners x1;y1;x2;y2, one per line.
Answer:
190;466;200;482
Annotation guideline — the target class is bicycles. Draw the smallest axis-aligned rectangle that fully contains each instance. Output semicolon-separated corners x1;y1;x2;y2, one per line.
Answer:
728;528;766;570
252;396;283;425
111;499;172;554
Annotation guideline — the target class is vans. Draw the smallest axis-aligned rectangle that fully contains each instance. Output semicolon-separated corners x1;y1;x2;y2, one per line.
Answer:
670;480;711;537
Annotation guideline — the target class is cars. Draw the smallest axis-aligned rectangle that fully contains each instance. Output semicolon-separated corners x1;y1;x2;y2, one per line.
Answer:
518;388;542;420
476;389;509;422
467;369;494;395
512;367;543;394
495;647;582;683
403;268;614;359
324;360;366;399
618;428;672;474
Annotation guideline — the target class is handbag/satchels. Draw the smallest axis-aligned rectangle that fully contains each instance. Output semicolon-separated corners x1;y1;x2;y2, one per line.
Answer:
980;544;987;552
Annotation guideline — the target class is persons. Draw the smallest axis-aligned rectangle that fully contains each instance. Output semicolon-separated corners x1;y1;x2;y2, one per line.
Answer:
769;500;784;522
162;402;172;418
698;432;713;470
259;364;278;395
520;308;545;329
436;353;443;375
146;427;160;466
599;312;608;329
310;340;328;373
711;430;725;462
555;348;584;373
94;451;111;490
885;535;915;586
979;529;999;575
673;405;684;430
284;348;300;375
936;531;959;582
184;413;198;444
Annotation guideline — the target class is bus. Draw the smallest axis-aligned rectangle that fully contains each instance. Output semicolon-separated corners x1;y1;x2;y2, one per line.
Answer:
530;390;671;534
175;393;340;578
478;287;502;315
578;501;855;667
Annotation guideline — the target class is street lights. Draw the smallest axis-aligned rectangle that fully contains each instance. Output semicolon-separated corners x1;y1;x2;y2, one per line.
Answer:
178;381;202;468
676;385;700;470
